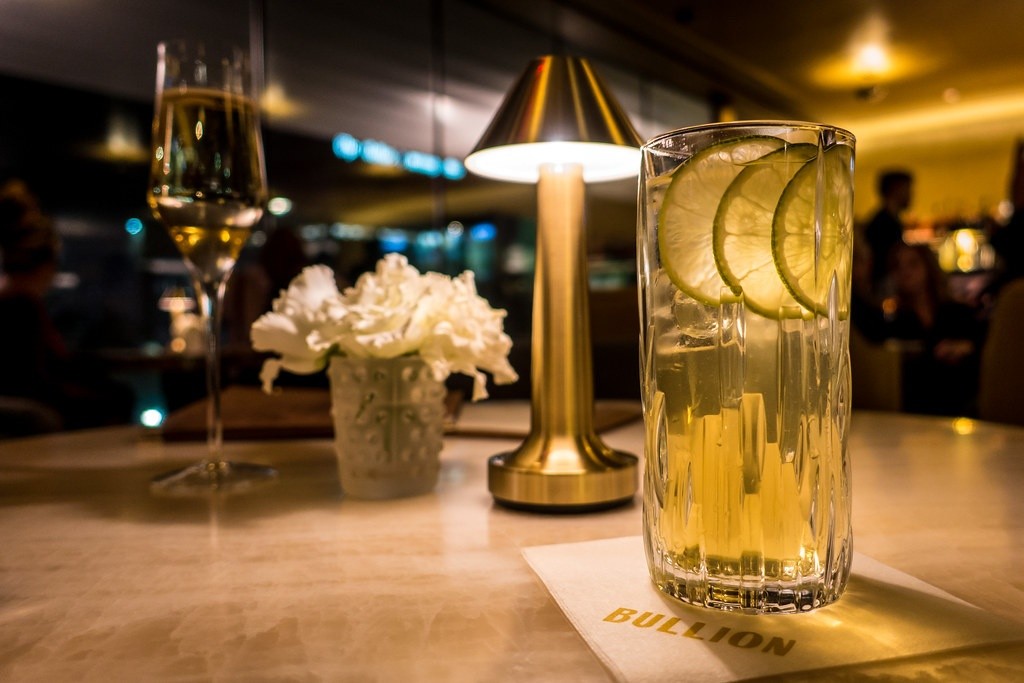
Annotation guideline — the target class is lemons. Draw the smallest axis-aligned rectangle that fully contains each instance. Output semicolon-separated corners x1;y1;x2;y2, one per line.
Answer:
657;136;853;321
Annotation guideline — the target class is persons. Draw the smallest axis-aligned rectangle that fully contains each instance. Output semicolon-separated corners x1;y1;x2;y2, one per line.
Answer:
979;280;1024;427
0;180;72;442
862;171;912;256
870;245;988;417
850;222;902;415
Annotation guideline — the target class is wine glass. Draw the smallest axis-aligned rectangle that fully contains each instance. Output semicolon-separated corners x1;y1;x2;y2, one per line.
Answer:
154;38;283;500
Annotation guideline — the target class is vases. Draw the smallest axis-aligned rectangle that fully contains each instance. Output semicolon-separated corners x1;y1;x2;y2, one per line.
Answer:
326;352;446;501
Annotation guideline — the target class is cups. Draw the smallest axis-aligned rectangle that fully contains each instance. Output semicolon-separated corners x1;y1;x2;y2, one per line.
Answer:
636;119;856;615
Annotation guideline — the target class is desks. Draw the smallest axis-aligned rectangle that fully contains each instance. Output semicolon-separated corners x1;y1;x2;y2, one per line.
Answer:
0;401;1024;683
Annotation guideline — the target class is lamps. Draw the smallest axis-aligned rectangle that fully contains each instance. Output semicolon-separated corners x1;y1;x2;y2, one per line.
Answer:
462;56;650;513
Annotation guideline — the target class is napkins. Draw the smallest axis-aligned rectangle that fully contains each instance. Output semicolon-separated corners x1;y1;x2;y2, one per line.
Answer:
518;532;1024;683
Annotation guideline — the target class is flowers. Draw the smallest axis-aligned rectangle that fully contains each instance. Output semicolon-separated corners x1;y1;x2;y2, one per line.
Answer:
252;251;518;401
